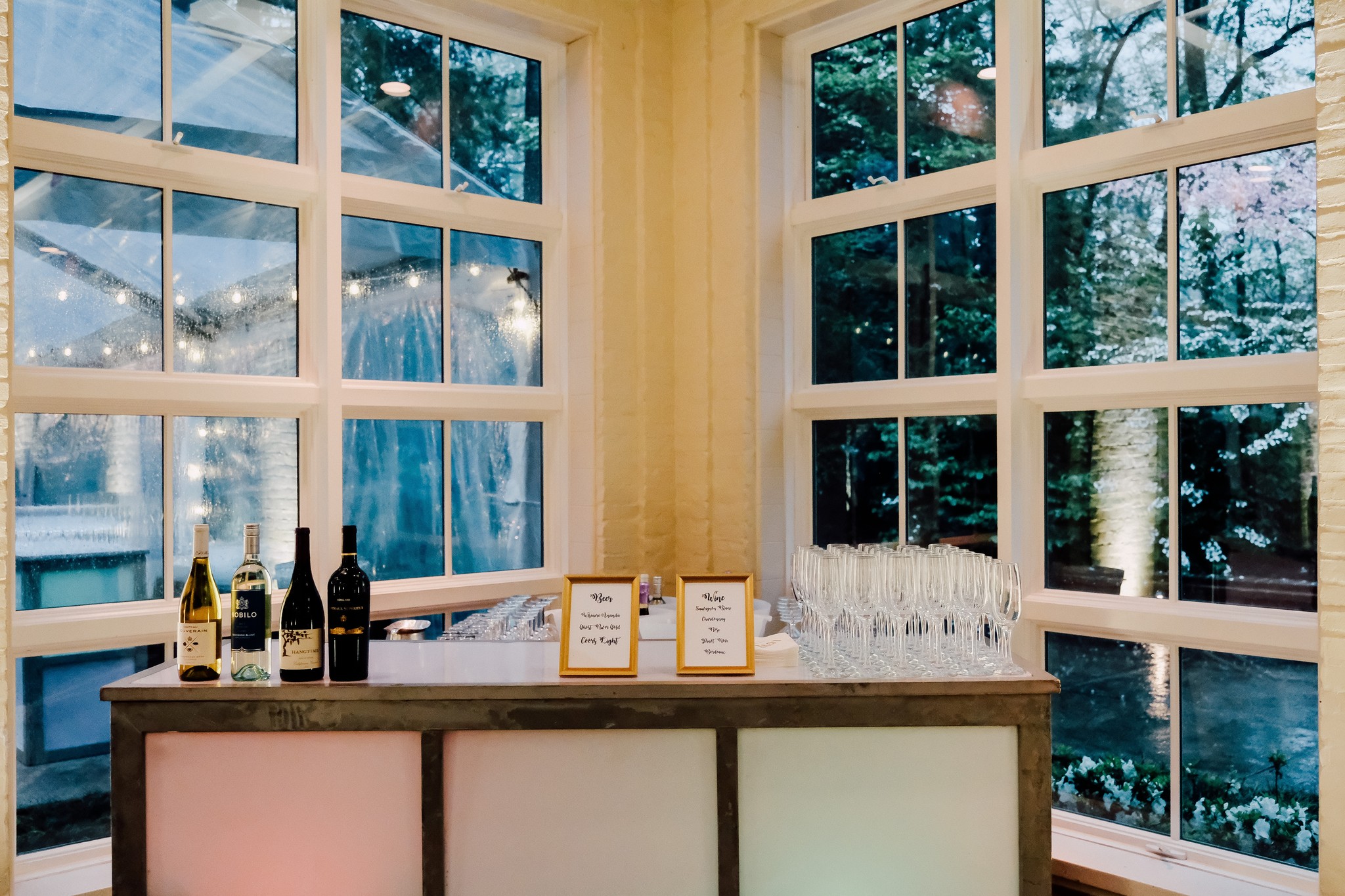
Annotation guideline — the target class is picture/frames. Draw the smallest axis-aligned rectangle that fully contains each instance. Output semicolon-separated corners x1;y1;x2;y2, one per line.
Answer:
558;572;642;679
674;571;757;678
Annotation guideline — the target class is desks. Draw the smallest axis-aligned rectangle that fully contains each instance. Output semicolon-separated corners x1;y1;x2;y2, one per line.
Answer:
94;637;1067;896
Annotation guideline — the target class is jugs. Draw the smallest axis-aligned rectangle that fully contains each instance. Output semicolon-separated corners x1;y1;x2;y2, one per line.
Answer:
384;619;431;640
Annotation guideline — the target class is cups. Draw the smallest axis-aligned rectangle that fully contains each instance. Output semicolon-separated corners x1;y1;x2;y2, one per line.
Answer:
754;598;771;637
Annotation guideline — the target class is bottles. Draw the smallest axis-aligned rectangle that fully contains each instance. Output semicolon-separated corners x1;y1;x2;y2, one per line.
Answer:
649;576;666;605
327;525;370;682
640;573;649;583
231;523;272;682
279;527;325;682
639;583;650;616
177;524;222;682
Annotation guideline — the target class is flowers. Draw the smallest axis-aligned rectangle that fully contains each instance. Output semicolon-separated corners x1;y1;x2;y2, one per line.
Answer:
1044;748;1320;874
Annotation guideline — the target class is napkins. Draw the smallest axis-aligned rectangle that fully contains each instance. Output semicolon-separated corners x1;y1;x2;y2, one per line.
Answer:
753;631;800;670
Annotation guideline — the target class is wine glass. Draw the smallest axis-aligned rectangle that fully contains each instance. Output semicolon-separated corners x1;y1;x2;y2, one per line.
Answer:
858;543;922;678
791;544;849;679
897;545;958;677
777;597;802;642
968;556;1026;676
827;544;885;679
928;544;992;676
438;594;560;642
